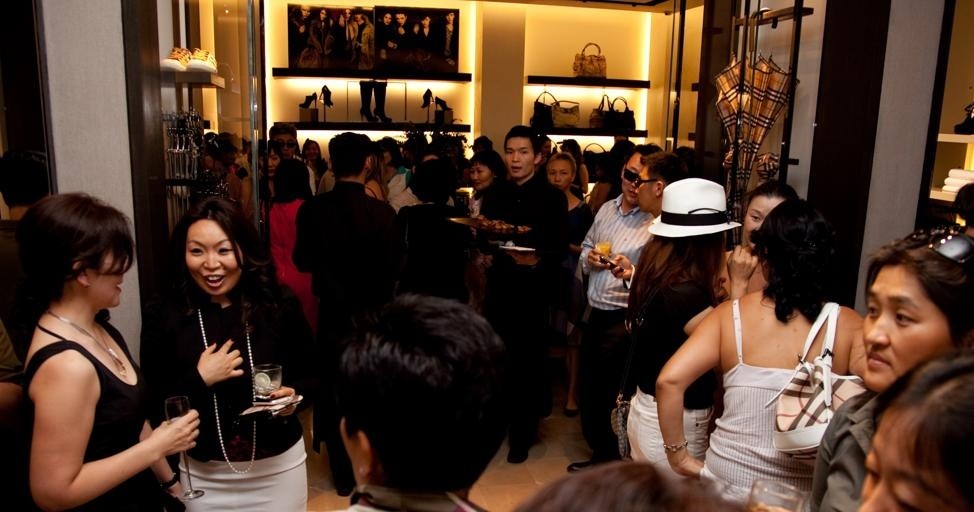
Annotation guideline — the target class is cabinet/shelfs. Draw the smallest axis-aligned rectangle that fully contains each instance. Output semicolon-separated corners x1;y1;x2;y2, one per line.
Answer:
927;132;974;202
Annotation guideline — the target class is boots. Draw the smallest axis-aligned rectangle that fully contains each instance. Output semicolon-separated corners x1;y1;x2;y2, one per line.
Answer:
373;81;392;122
358;80;379;121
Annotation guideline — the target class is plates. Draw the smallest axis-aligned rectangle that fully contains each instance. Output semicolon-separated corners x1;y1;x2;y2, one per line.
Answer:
499;245;537;253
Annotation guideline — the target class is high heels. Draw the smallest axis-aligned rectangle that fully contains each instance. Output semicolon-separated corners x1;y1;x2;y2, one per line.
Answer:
298;92;318;109
435;96;453;110
420;89;434;109
319;84;334;108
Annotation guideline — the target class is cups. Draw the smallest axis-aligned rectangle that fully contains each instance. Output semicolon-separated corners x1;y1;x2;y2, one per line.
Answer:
745;478;806;512
594;242;611;264
250;361;284;402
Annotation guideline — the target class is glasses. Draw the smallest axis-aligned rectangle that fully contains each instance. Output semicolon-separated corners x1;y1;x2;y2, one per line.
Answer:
623;163;638;183
906;225;973;265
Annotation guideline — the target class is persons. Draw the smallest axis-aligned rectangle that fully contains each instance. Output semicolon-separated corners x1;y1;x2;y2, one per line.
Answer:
2;127;973;512
291;5;456;69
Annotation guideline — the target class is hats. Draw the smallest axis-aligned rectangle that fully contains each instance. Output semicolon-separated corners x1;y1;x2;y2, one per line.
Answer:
648;177;743;238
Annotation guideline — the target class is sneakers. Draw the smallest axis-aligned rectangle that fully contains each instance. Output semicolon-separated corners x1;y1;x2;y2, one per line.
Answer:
160;46;219;74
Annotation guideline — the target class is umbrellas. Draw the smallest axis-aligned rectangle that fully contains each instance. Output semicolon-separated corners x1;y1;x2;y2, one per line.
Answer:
713;8;801;244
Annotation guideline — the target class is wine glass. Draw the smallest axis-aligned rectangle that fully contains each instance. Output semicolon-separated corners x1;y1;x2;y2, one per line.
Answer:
163;396;204;503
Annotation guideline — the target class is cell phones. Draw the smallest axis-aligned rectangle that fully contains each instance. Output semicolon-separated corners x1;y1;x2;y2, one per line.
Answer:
600;256;625;272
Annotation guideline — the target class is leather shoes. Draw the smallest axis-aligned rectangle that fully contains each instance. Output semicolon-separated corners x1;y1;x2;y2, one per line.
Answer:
567;459;593;473
507;444;529;464
564;401;578;418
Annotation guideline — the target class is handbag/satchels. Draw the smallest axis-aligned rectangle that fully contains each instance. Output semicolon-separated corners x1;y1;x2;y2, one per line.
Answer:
573;42;607;78
582;143;609;182
533;91;580;128
589;95;636;130
767;302;866;467
